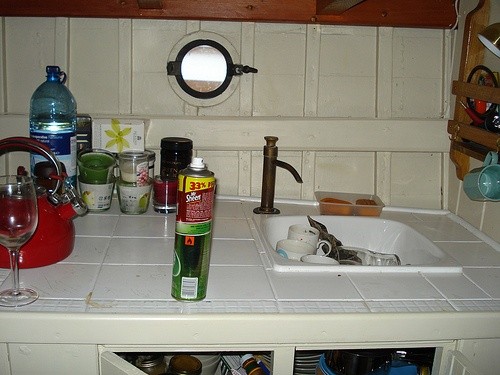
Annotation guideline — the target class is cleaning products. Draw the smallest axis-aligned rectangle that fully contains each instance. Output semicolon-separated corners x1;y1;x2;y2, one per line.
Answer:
28;64;79;189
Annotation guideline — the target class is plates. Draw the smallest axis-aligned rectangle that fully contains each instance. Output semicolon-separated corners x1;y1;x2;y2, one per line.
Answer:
319;353;417;375
263;352;322;374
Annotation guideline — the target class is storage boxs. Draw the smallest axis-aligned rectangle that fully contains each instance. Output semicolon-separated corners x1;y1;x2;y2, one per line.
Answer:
314;190;384;217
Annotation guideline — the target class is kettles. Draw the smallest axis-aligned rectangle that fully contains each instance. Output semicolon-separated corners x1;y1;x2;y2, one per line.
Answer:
0;136;88;269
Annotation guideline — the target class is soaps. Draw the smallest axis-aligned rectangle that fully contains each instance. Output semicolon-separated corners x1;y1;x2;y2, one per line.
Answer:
320;197;353;216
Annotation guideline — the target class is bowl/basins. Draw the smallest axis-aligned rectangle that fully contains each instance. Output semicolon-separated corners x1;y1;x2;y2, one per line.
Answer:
164;355;222;375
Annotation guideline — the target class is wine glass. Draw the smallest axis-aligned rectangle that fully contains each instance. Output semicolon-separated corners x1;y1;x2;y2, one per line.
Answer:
0;174;38;307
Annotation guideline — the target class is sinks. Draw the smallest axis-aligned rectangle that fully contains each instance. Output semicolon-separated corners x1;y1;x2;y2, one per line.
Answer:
355;199;378;216
250;212;463;275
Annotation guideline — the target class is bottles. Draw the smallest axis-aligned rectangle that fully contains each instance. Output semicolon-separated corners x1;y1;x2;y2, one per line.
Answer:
240;353;268;375
144;151;156;182
474;74;488;118
166;355;202;375
29;66;77;190
485;103;500;134
170;157;216;301
137;352;166;375
159;137;193;181
119;150;148;186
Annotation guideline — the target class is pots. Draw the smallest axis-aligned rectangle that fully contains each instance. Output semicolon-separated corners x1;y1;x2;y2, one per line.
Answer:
325;347;436;375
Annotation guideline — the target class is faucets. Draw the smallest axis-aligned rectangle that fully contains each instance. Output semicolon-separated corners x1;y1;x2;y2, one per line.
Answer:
252;135;303;214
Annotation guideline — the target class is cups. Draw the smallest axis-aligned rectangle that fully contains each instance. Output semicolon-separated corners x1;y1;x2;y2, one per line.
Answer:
117;181;153;215
76;149;117;184
276;239;315;262
301;255;340;265
79;181;114;213
462;151;500;201
287;224;332;258
153;176;179;214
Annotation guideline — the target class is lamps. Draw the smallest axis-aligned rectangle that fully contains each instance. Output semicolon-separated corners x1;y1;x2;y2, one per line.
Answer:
476;23;500;58
